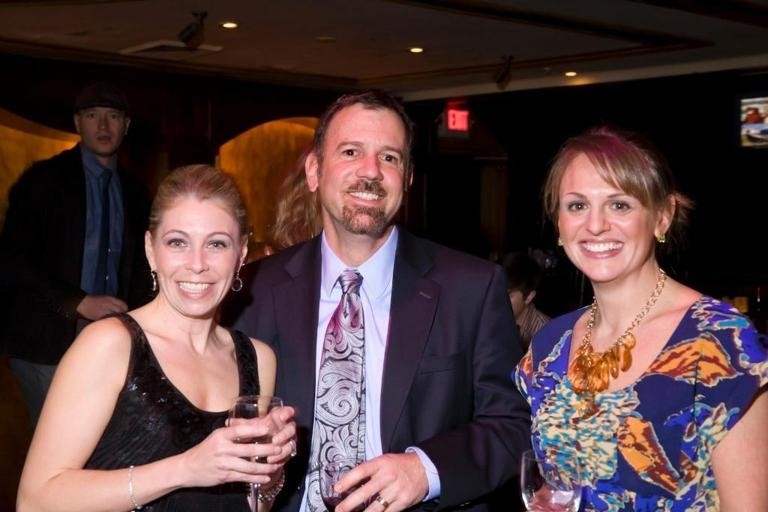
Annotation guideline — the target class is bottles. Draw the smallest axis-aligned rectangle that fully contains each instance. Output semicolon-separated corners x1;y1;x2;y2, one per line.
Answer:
754;287;763;320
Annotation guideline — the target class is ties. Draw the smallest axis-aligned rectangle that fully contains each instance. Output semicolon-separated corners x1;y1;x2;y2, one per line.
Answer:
304;269;366;512
94;167;114;296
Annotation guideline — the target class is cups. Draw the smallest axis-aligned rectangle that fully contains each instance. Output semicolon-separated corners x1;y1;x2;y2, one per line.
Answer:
320;458;365;512
520;448;583;511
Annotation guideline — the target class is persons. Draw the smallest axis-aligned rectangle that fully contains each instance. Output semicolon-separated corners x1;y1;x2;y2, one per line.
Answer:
462;231;499;264
216;89;535;512
506;125;768;512
499;261;553;358
15;165;302;512
0;85;160;437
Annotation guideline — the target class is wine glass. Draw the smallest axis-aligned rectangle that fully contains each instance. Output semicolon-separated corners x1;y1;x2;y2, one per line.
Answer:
229;395;284;512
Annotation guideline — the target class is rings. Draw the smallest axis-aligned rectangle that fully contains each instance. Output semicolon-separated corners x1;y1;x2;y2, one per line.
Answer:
378;495;389;508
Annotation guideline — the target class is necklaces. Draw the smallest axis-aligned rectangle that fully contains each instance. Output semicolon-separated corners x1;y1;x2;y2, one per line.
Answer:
565;267;669;397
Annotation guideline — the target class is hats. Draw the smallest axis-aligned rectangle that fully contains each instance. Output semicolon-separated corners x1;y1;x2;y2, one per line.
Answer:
75;83;129;112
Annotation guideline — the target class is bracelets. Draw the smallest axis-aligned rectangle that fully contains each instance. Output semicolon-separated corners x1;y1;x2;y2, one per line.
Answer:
126;463;143;511
